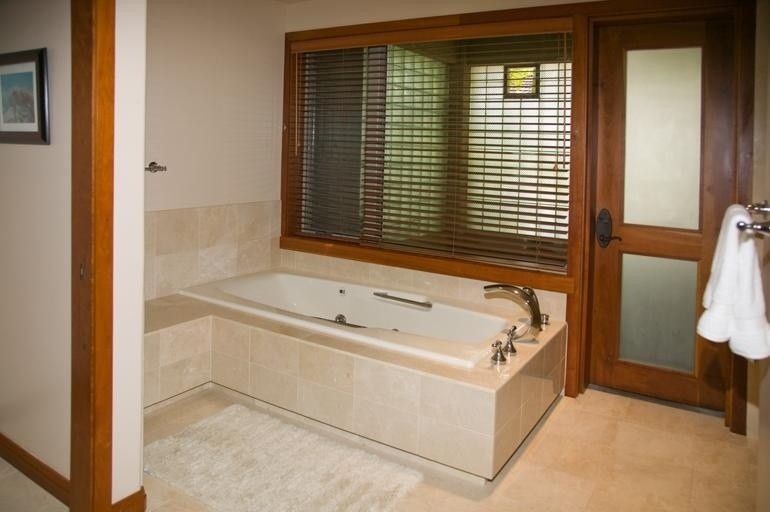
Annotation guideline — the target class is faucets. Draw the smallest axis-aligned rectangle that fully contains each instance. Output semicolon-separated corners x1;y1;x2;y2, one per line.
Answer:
483;283;541;342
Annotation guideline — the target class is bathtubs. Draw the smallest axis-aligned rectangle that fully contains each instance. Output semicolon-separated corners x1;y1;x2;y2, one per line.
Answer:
179;266;532;370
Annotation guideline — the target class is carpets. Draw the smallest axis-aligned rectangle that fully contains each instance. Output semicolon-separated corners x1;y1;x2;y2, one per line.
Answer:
142;401;424;512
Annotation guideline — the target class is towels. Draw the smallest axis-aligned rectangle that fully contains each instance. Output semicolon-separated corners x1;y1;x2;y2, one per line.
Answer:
694;201;770;363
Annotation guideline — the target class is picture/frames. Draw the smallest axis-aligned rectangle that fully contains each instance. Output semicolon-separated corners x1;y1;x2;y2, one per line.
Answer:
0;45;53;145
502;62;541;99
